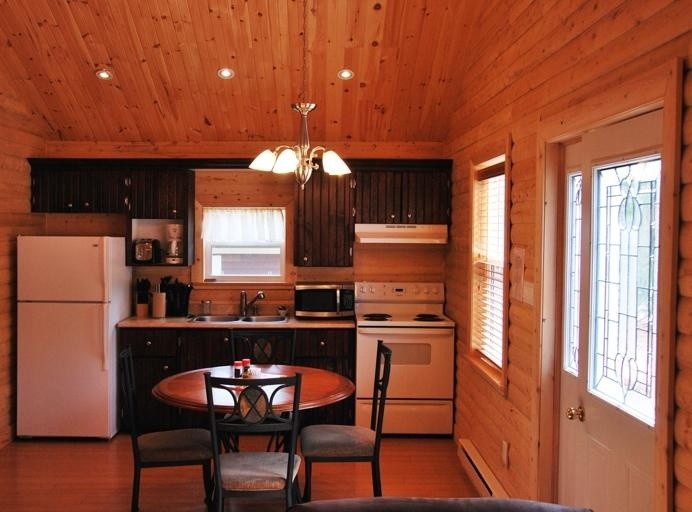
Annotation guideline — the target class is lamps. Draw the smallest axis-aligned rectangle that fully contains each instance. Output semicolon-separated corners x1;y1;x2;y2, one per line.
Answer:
249;102;352;190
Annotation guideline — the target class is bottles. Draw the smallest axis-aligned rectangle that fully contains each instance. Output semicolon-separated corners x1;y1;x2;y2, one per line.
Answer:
233;361;243;377
242;359;250;378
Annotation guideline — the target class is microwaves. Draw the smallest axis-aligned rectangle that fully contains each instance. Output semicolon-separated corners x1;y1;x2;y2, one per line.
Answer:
294;283;354;319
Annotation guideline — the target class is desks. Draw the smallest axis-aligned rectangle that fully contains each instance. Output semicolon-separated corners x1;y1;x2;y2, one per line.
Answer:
152;363;356;512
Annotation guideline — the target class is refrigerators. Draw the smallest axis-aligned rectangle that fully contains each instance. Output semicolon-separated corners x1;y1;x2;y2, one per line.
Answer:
16;234;132;440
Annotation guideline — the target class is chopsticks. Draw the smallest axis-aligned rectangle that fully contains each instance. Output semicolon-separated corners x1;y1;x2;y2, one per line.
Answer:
136;278;151;304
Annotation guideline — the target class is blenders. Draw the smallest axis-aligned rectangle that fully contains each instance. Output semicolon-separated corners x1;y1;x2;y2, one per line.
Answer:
165;224;183;264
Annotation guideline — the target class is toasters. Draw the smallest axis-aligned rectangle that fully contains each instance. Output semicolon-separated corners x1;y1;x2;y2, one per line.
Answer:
132;238;160;264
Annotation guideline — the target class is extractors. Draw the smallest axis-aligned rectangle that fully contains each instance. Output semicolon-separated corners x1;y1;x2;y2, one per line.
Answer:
352;223;448;244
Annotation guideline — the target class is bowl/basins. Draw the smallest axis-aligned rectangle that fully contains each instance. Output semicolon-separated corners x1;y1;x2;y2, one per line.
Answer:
279;309;288;316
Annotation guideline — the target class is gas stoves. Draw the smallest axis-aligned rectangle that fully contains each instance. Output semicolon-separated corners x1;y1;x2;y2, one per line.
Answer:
355;282;455;326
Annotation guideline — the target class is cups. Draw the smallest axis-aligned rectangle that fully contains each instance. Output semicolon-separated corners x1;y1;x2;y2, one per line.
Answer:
203;300;211;315
249;368;262;377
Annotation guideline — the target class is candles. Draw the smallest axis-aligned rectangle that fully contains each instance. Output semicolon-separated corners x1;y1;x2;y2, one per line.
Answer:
234;361;242;367
242;358;250;367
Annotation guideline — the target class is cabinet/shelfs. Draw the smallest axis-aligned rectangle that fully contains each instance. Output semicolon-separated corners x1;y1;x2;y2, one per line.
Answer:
293;169;353;267
30;165;127;214
117;328;233;435
231;329;356;425
352;170;452;225
126;167;196;267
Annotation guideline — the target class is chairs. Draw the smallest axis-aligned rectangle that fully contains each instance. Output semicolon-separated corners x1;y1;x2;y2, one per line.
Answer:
299;339;392;502
120;343;214;512
202;371;302;512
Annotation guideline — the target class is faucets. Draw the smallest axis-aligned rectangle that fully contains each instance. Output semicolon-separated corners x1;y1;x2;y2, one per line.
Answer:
239;290;265;316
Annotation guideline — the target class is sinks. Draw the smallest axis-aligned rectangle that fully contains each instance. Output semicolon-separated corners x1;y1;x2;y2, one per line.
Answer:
239;315;288;324
188;314;240;323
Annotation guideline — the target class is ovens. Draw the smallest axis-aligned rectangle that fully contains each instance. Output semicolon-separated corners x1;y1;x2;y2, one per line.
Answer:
356;327;455;435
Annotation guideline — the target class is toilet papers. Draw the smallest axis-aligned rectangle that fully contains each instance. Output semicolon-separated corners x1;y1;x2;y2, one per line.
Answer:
154;292;167;317
